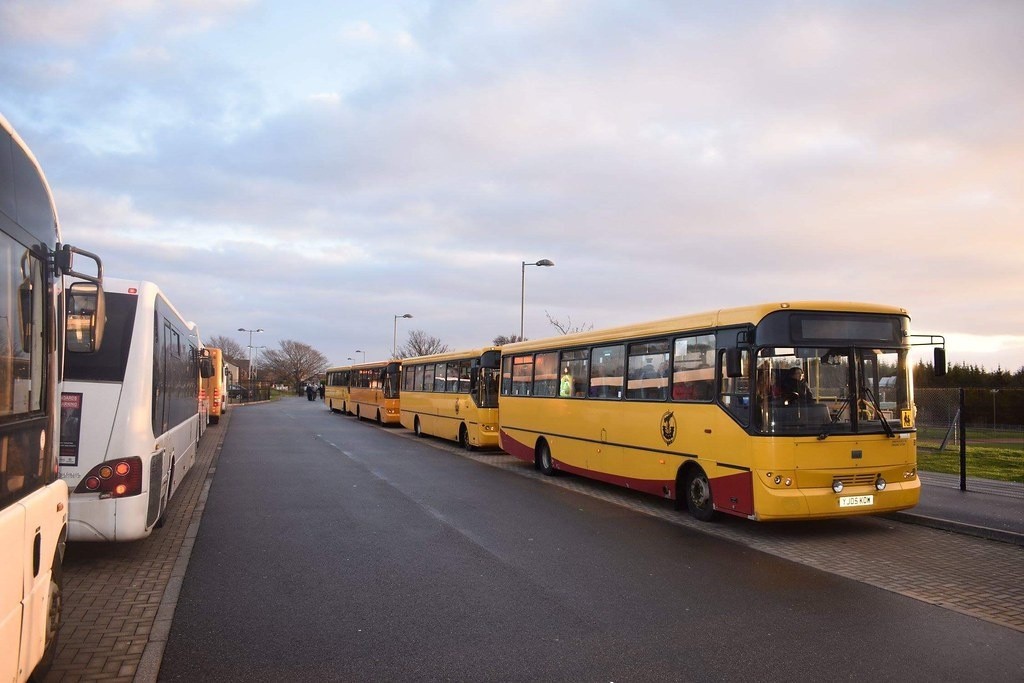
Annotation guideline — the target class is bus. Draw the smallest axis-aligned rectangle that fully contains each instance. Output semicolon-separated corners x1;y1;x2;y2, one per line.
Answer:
61;273;215;544
351;360;402;427
0;112;105;682
497;301;946;522
324;366;351;416
221;364;233;414
201;348;229;425
398;346;502;451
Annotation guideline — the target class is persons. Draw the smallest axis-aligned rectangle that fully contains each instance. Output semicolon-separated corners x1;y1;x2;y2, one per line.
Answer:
785;367;816;406
305;382;325;401
759;369;780;409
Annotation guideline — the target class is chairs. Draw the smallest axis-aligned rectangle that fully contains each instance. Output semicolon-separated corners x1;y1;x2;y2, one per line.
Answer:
511;370;729;400
758;368;788;397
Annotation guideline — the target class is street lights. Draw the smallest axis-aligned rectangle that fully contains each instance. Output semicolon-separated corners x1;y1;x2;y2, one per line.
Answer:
238;328;264;380
347;358;355;364
520;259;555;342
393;314;415;360
246;345;267;379
356;350;365;363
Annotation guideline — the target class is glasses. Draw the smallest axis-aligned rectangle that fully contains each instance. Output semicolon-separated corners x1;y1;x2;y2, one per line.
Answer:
795;372;805;376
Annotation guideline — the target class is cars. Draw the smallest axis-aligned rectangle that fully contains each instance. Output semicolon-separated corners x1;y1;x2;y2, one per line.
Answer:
227;384;253;400
